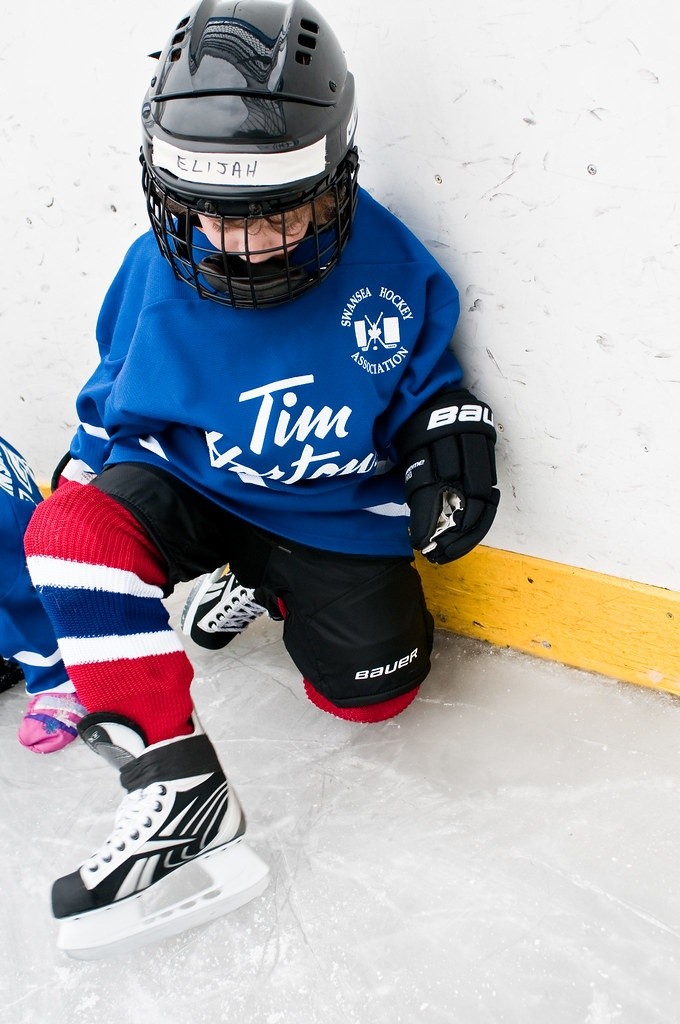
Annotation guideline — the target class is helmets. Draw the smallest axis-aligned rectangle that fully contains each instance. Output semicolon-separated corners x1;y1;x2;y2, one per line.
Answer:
143;0;356;218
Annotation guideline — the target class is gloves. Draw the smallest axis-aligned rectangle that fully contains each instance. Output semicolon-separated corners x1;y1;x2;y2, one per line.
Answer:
405;392;500;564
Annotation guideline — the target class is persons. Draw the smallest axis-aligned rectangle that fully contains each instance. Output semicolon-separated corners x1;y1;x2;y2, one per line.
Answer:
20;0;504;961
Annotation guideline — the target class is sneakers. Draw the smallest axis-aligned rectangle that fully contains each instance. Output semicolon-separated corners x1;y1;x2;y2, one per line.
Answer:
50;709;269;955
179;562;270;649
